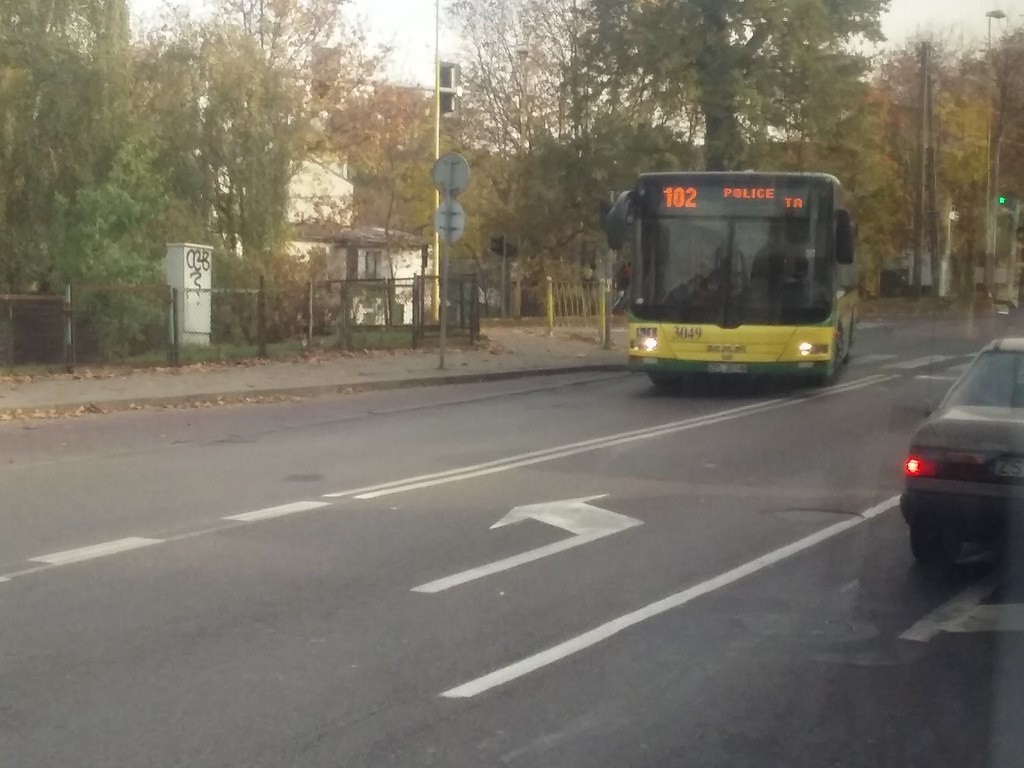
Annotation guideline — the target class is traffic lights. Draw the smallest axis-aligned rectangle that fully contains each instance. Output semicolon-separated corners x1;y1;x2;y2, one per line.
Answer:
999;195;1006;205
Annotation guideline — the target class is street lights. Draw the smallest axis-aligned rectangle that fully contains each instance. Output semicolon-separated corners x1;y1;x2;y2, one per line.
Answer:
983;9;1006;309
506;45;531;318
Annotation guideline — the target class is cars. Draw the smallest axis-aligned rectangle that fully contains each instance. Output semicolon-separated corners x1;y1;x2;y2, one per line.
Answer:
900;337;1024;560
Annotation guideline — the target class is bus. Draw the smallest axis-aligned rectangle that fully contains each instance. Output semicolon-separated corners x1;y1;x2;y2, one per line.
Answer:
603;171;864;390
